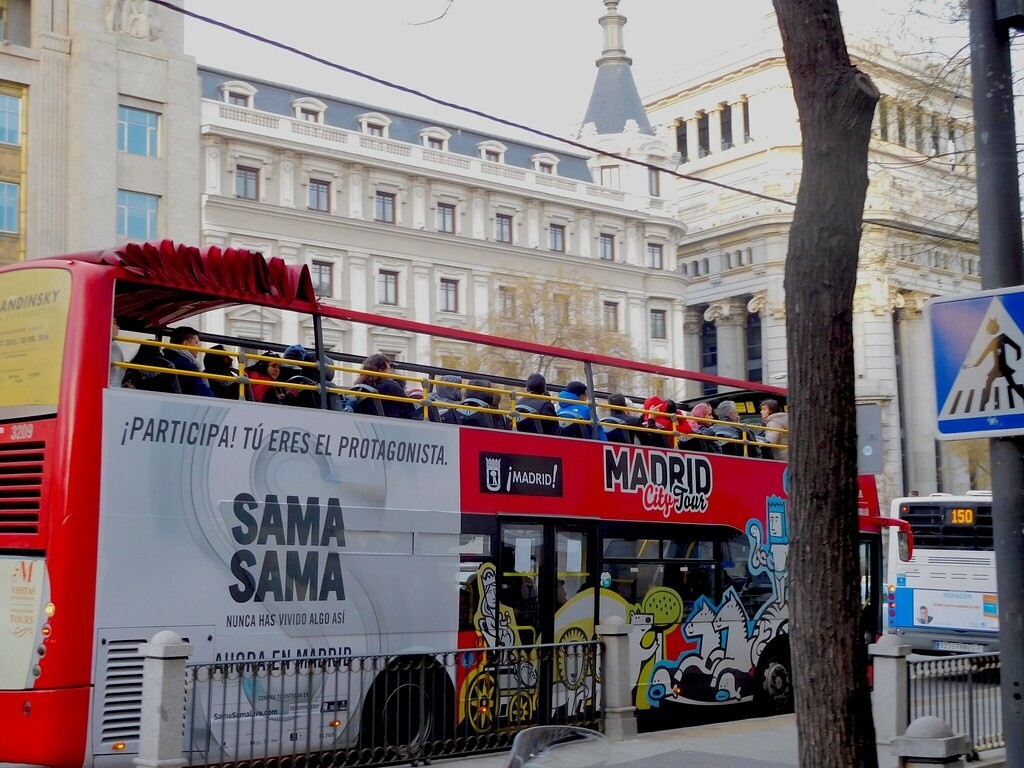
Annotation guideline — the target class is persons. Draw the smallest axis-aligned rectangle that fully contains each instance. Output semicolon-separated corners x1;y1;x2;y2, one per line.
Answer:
109;317;790;462
919;606;933;624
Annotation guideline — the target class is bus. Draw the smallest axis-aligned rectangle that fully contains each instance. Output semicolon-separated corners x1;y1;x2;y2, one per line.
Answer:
0;242;913;768
888;489;1002;658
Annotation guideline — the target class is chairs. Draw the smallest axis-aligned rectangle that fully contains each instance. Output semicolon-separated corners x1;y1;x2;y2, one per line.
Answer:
503;405;545;433
281;375;321;409
344;383;385;416
202;367;256;401
408;391;440;422
557;411;589;439
123;357;181;395
639;420;670;447
454;398;495;428
678;428;707;451
716;431;741;456
755;434;774;460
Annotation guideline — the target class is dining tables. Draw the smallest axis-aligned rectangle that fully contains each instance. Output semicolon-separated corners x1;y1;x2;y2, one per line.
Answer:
600;416;631;444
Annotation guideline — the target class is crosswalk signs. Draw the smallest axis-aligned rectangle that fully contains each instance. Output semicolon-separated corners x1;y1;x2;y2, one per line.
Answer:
925;282;1024;443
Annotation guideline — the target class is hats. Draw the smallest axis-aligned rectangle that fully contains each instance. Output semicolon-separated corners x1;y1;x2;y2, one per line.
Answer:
566;381;586;397
608;393;625;407
284;345;305;360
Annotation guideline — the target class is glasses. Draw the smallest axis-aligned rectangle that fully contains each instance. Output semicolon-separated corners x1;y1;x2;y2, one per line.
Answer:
268;363;280;369
